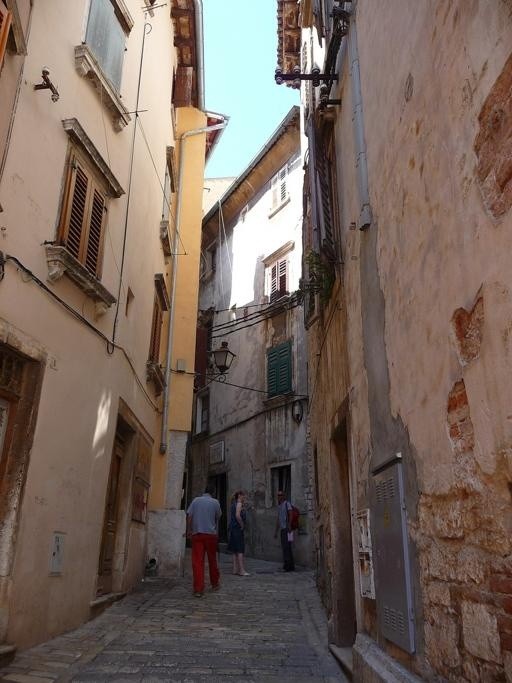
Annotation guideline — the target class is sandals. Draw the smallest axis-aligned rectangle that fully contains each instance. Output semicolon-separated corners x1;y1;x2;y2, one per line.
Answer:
238;571;251;577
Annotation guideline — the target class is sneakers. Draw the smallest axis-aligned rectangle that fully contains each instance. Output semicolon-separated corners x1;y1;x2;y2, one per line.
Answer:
193;591;203;597
211;582;220;591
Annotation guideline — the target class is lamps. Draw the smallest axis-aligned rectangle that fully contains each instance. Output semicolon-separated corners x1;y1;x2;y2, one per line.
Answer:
194;342;237;394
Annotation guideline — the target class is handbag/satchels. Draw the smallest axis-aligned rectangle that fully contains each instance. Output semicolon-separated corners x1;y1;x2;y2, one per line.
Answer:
287;506;300;530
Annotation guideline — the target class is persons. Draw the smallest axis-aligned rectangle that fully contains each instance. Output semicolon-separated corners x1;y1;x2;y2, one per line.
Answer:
184;484;223;597
228;488;252;575
272;489;295;573
